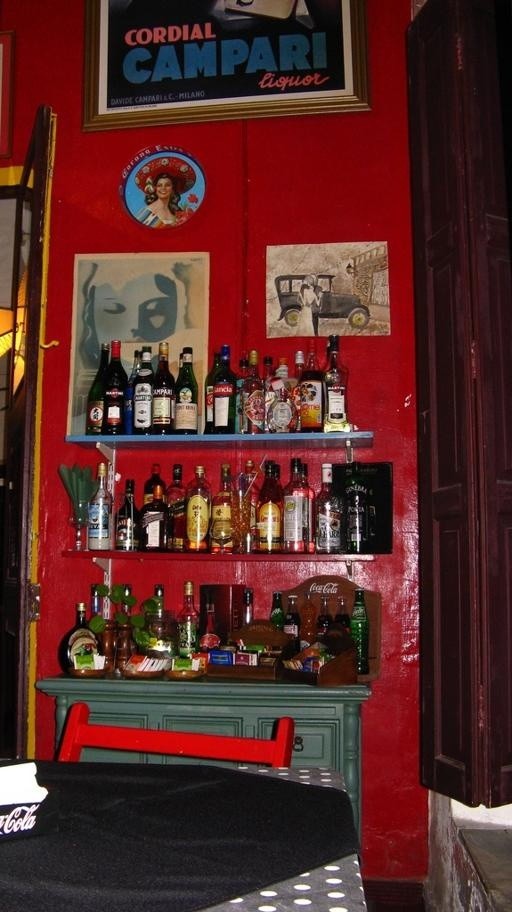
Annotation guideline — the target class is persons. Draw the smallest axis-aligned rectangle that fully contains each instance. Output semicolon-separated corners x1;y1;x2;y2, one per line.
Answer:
134;156;196;228
81;259;177;371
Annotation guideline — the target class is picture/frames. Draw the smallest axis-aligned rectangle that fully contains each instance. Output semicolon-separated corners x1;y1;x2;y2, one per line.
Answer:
80;0;371;134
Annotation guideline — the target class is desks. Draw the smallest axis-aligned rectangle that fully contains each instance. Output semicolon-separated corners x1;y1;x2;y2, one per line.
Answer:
2;758;369;912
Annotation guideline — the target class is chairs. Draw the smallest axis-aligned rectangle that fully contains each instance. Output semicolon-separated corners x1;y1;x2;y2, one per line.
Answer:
56;698;297;769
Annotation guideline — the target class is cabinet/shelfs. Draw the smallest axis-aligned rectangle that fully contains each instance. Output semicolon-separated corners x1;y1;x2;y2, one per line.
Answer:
30;674;374;859
51;432;377;559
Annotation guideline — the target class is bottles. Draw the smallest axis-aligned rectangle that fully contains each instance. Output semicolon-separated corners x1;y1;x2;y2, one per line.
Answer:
84;332;349;434
57;578;371;682
89;460;373;553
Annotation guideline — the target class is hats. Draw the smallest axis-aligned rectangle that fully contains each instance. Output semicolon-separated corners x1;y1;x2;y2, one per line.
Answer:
134;155;198;198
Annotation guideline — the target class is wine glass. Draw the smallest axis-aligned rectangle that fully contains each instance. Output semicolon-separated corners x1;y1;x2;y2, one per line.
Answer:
64;500;90;551
